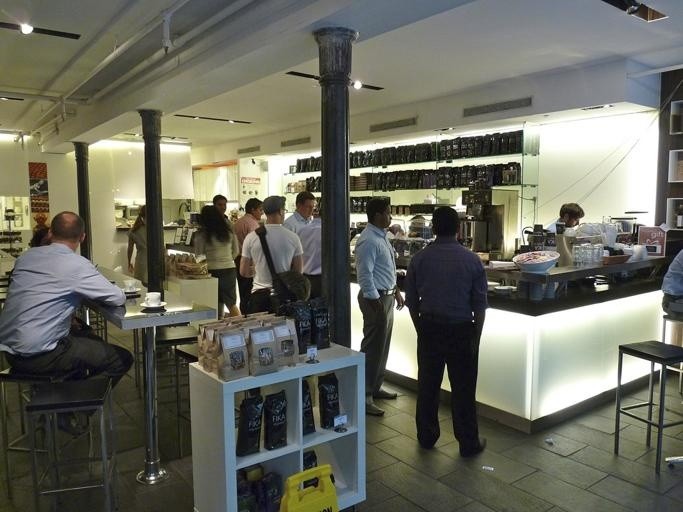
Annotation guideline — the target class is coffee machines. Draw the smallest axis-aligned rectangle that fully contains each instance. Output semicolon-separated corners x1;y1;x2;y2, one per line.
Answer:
461;215;488;253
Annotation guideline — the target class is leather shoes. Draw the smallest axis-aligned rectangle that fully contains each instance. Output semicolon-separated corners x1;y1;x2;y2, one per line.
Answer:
462;436;487;457
366;403;385;416
373;389;397;400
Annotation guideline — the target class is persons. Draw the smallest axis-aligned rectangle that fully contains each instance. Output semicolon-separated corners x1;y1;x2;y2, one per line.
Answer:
194;189;321;316
30;227;52;247
128;205;149;288
662;249;683;323
405;206;489;457
0;210;127;437
356;197;404;416
545;204;584;245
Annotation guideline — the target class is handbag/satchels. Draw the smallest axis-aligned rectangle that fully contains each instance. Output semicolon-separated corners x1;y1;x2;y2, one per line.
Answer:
272;270;312;301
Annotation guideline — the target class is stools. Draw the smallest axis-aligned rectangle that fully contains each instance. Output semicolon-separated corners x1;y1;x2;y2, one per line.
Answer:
1;249;217;511
658;311;682;398
612;335;681;479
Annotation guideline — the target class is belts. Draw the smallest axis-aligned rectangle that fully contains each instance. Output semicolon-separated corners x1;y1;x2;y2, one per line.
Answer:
379;288;397;296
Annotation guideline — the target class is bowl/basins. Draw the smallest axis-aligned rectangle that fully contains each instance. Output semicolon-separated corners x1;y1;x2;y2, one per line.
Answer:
603;254;632;264
513;251;560;273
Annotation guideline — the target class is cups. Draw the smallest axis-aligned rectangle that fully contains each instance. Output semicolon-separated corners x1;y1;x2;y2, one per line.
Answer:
573;243;604;269
633;245;648;262
145;292;161;306
124;279;137;292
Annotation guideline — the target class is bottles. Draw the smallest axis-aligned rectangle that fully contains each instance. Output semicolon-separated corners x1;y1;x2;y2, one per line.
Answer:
530;224;559;267
604;241;630;257
676;205;683;229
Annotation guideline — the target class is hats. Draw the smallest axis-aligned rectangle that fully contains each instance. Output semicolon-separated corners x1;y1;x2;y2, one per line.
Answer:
263;195;286;212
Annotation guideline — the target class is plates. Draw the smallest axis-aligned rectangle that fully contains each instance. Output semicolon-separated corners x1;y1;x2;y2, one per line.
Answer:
486;279;554;303
122;288;141;293
141;301;167;308
350;175;367;191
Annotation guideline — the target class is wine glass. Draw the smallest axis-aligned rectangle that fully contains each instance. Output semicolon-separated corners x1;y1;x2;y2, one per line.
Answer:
127;217;136;227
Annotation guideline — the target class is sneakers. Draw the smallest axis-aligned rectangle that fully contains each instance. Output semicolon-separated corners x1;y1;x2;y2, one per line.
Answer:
38;412;92;435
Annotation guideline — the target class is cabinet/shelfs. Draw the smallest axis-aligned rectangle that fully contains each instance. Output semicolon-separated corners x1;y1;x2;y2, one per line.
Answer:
664;99;682;233
280;122;537;245
184;339;370;511
